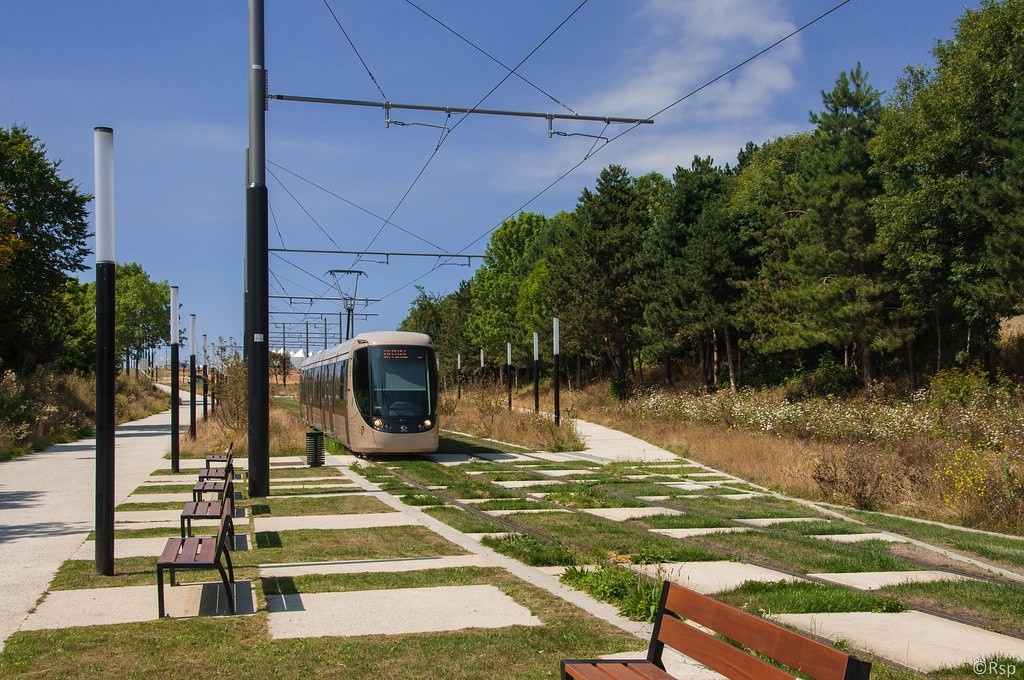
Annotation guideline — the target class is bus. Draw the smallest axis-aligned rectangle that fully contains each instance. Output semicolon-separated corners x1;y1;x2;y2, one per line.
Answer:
298;330;442;456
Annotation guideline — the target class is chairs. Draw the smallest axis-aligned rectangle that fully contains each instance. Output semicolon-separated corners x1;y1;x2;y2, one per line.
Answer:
192;459;233;503
198;449;233;482
180;473;234;550
156;498;234;618
206;442;234;480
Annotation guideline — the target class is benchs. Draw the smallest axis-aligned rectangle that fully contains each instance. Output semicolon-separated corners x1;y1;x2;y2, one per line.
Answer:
559;581;871;678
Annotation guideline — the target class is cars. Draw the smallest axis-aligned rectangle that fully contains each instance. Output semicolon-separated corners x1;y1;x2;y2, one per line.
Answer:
188;374;203;383
